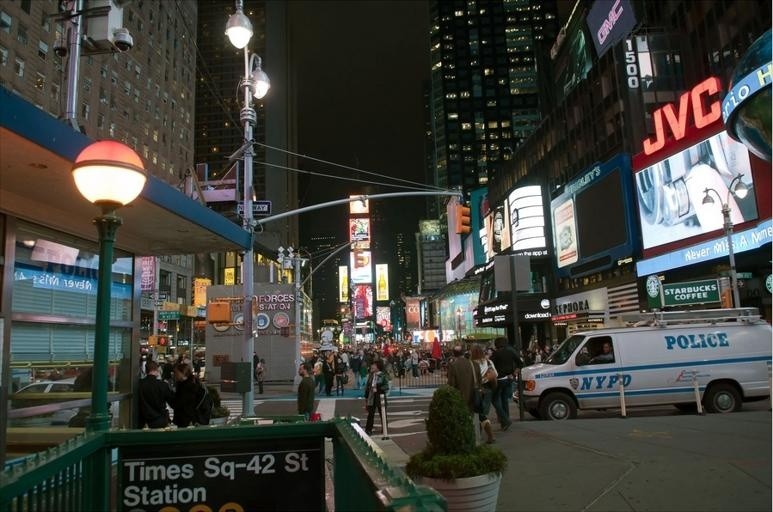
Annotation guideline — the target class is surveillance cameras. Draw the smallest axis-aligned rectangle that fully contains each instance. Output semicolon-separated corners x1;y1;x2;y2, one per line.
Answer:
54;39;67;58
114;32;134;51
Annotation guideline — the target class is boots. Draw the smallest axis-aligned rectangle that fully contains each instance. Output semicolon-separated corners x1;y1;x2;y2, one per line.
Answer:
481;419;497;444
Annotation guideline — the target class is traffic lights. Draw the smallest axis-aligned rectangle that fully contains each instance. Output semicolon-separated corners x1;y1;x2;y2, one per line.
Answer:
355;246;364;269
453;201;472;236
156;335;169;346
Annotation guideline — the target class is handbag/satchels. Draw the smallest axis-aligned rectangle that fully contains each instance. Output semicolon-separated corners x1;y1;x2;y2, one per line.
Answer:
486;367;500;393
342;375;349;385
471;386;490;415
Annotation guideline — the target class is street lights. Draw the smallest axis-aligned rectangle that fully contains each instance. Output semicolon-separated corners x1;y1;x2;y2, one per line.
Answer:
275;243;295;283
65;136;154;433
220;0;272;425
456;307;463;338
700;173;751;307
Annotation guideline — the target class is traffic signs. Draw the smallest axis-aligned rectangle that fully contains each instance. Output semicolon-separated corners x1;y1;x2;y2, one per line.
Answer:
145;292;181;322
734;272;752;279
235;199;271;217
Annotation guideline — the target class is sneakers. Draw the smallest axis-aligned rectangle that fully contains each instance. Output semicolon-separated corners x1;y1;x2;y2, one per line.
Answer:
500;418;513;431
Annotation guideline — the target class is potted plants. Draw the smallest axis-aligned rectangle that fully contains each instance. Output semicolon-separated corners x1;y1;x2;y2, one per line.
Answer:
407;384;507;512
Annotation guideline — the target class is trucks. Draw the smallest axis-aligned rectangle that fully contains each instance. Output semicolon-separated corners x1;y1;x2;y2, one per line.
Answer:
506;305;771;423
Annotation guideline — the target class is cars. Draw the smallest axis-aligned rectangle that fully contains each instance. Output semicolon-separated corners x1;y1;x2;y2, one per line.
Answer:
142;338;204;374
8;378;119;428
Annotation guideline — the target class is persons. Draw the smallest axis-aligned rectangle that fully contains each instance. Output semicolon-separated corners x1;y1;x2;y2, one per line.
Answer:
174;364;200;428
311;335;550;410
138;361;175;428
253;352;258;378
364;361;389;441
256;358;265;394
157;349;202;391
471;345;499;444
589;341;613;363
298;363;315;420
490;337;523;430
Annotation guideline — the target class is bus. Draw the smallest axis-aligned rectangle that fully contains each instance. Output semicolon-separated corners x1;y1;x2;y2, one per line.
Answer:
353;322;376;344
